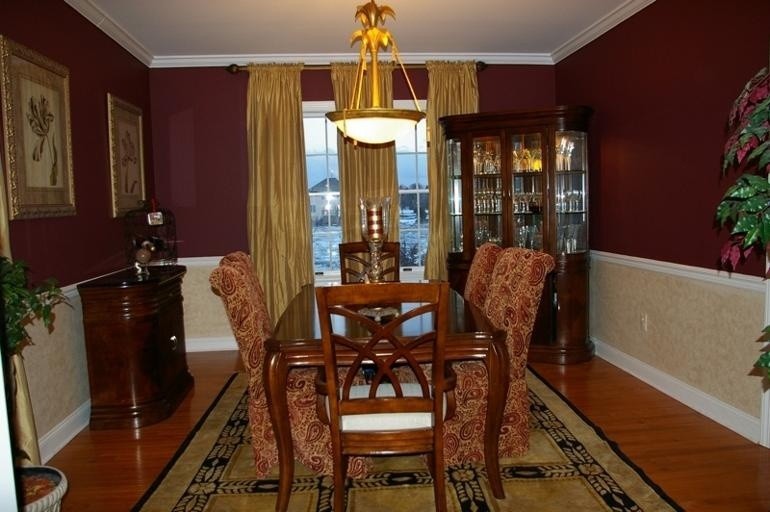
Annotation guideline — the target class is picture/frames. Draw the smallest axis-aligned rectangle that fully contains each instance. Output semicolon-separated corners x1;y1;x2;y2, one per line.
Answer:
107;92;146;218
0;33;76;221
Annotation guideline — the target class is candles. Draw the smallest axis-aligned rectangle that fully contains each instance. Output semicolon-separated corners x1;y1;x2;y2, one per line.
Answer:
367;204;383;240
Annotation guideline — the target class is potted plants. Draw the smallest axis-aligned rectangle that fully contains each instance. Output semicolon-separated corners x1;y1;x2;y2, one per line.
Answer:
0;256;75;512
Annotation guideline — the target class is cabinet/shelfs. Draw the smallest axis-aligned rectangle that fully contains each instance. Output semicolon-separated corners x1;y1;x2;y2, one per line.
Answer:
77;265;194;430
439;106;595;365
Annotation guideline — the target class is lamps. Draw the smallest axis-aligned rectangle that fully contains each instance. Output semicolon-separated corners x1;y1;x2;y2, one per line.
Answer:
326;0;426;146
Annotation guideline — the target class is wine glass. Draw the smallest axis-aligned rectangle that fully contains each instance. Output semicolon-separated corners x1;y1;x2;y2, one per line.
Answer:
518;223;581;255
473;148;543;176
474;187;582;214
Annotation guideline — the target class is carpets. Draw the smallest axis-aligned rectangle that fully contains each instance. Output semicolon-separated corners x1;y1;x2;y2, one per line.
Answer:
131;364;686;512
